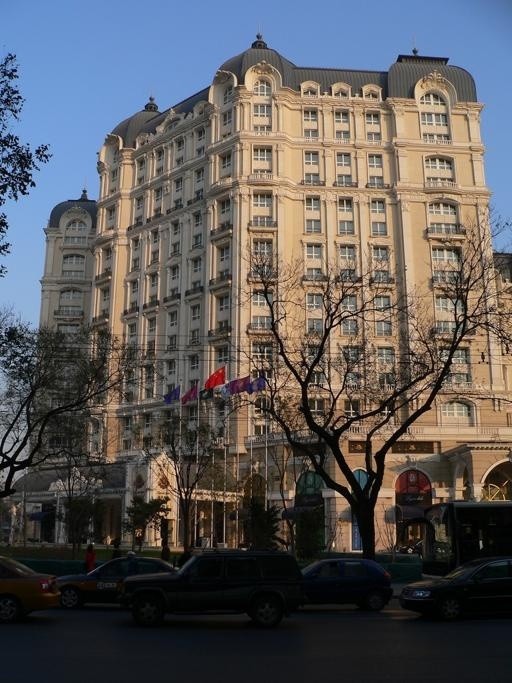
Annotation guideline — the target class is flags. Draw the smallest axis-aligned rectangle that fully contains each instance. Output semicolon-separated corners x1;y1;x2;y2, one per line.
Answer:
219;385;228;402
225;375;249;394
247;376;265;393
163;383;181;404
205;366;225;386
181;385;198;403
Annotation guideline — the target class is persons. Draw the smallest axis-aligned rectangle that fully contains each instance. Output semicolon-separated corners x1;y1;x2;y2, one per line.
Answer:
112;541;122;565
161;539;170;561
127;550;138;576
178;544;196;567
84;543;97;572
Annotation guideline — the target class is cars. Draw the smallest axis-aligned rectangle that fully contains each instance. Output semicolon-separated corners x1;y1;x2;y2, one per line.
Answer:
400;539;423;553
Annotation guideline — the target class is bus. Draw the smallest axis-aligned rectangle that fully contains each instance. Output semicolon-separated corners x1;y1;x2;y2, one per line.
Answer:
422;501;512;577
